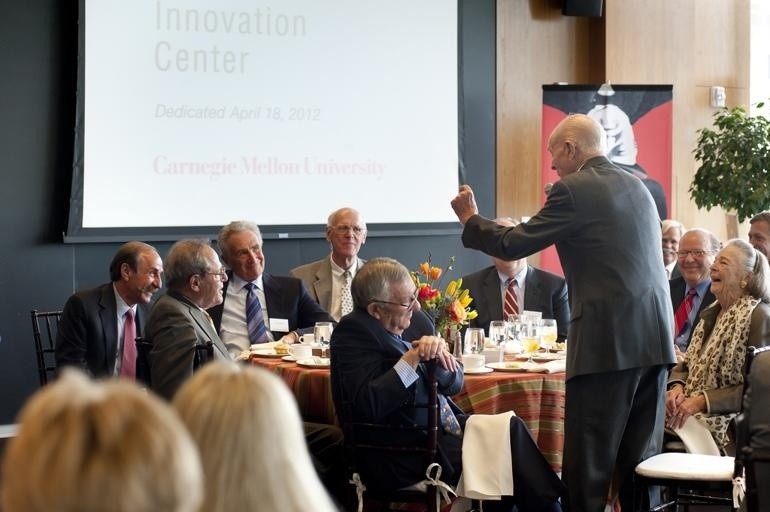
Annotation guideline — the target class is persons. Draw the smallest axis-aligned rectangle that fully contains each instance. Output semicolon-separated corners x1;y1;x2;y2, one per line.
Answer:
143;239;359;512
54;239;164;391
170;359;343;512
1;364;207;512
449;113;679;511
458;213;570;344
657;210;770;512
202;219;339;366
328;255;571;510
544;102;668;229
288;207;369;325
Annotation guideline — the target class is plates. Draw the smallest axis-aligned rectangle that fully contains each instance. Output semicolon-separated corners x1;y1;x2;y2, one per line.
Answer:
250;341;331;369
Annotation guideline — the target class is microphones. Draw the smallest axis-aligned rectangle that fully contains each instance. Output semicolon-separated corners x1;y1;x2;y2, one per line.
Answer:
544;183;553;195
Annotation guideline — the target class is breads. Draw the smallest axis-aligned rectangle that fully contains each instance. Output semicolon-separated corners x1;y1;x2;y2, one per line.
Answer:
274;344;289;354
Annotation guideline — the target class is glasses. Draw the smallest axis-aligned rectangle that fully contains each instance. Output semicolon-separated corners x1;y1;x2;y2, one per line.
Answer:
674;248;714;259
207;266;226;282
328;223;364;236
371;286;420;312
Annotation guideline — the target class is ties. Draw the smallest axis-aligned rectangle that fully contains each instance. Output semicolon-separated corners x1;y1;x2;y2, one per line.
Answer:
120;309;137;386
673;287;697;340
242;282;269;345
502;278;519;323
340;270;355;319
393;335;463;442
203;312;219;338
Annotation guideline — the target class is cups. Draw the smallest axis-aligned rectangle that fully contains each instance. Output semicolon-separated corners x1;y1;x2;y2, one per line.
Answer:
287;319;334;359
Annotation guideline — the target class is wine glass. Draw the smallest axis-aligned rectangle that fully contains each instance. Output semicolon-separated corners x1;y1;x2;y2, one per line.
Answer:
461;308;566;375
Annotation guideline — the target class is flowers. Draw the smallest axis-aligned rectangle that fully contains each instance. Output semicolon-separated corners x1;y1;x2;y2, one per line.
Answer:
408;249;478;336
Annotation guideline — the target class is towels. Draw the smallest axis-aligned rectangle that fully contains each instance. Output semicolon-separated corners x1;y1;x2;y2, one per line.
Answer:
525;356;566;374
455;409;518;500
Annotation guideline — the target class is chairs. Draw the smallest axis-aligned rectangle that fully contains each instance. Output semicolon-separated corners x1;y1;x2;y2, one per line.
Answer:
134;337;215;401
634;345;770;511
31;309;63;385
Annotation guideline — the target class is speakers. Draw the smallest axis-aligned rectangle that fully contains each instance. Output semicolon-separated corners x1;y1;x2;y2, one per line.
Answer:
561;0;603;17
